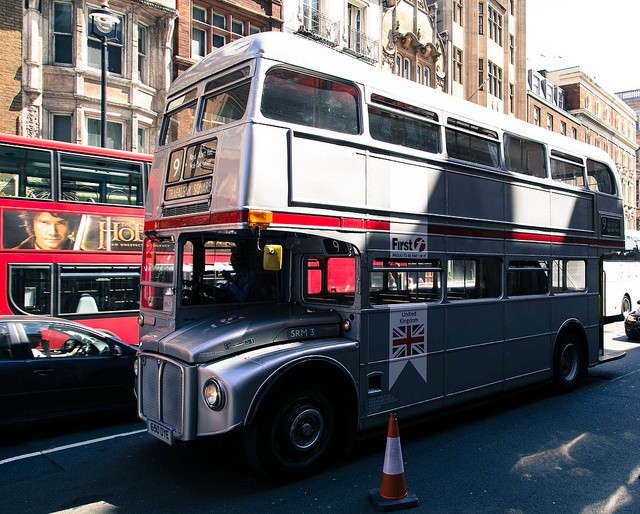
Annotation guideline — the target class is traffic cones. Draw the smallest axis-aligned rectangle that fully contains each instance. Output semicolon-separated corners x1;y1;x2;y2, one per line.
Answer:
369;413;419;512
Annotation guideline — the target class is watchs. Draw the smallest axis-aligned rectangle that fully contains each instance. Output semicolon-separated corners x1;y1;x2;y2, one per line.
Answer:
229;282;234;285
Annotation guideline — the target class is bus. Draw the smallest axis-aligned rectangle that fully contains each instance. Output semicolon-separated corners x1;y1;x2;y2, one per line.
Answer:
134;31;626;473
0;132;233;346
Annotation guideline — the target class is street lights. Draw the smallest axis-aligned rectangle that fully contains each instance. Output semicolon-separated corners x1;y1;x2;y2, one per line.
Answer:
89;4;121;146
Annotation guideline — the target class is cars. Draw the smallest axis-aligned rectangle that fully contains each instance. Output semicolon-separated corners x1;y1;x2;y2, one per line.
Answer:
0;314;141;435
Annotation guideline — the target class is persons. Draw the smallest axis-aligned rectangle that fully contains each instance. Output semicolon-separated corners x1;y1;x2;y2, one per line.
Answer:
25;324;84;359
221;252;255;302
14;210;81;251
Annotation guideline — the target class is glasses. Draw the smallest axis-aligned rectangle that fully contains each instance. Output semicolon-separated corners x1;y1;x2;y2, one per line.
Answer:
34;336;42;339
